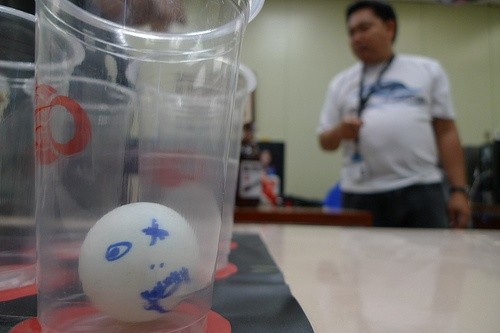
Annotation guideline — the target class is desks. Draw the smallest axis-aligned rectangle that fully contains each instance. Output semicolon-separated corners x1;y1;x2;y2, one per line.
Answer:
1;216;500;333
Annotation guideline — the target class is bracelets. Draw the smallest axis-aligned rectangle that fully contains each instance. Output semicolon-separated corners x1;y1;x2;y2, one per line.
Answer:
451;183;470;195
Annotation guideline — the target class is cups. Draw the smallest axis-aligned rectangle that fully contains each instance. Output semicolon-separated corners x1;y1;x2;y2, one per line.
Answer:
1;4;85;292
33;0;267;333
32;72;134;240
126;50;258;272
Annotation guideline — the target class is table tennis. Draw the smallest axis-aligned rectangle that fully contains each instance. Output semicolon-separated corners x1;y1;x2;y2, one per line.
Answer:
77;200;200;324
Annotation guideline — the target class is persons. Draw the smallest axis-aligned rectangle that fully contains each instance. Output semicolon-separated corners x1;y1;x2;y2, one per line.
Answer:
314;0;471;227
0;0;191;213
259;150;276;176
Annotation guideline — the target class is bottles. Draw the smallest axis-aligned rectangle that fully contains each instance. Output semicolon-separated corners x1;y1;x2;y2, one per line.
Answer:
236;121;263;205
262;166;281;205
